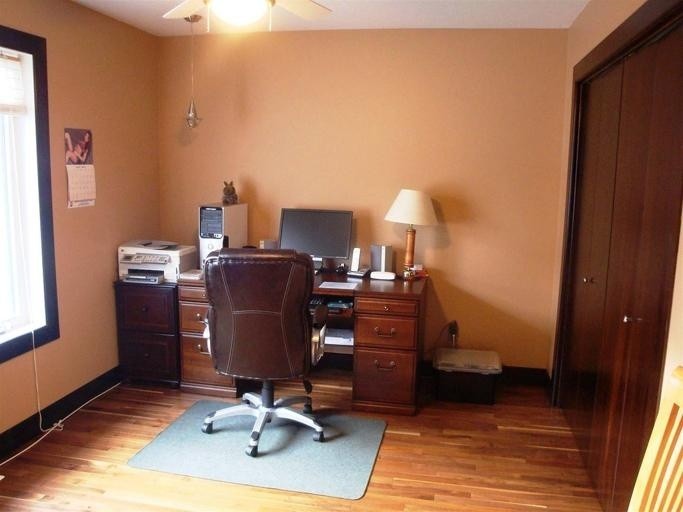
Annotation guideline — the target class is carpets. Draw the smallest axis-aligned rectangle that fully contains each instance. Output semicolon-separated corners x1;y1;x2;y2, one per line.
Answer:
126;399;387;499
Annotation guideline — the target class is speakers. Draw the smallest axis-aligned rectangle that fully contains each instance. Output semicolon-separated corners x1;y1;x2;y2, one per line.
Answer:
370;244;393;272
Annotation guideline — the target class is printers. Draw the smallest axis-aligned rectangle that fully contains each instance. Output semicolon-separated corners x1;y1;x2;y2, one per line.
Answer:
117;239;198;285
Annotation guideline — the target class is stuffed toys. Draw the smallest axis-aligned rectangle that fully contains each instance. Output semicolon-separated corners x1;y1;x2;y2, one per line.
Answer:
221;181;239;204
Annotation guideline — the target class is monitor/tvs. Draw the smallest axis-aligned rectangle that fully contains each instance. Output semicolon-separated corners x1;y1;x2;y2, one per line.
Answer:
277;208;353;275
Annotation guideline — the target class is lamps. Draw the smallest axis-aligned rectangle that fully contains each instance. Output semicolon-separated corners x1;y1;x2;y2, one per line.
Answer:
206;0;274;31
182;14;202;128
383;189;439;281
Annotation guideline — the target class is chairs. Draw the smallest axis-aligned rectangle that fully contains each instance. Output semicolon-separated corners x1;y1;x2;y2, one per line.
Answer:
202;248;328;456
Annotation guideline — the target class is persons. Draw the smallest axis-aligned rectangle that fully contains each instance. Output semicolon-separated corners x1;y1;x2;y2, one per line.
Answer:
64;129;91;164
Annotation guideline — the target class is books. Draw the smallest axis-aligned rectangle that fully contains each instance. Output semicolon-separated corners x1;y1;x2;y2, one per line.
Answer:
323;328;354;345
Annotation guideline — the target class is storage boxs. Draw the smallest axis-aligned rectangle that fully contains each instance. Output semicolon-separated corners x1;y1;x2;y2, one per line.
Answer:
431;348;503;405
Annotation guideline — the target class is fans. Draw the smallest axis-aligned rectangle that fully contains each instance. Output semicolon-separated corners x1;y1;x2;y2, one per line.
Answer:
162;0;330;20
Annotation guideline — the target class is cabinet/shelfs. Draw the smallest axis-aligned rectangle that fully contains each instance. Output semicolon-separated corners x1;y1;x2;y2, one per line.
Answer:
548;0;683;512
176;267;428;416
112;277;180;390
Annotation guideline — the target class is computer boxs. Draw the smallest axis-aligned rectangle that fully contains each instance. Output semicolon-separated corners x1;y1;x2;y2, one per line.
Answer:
198;202;248;270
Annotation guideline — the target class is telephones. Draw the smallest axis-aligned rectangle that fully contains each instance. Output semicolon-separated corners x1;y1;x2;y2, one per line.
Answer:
346;248;370;277
